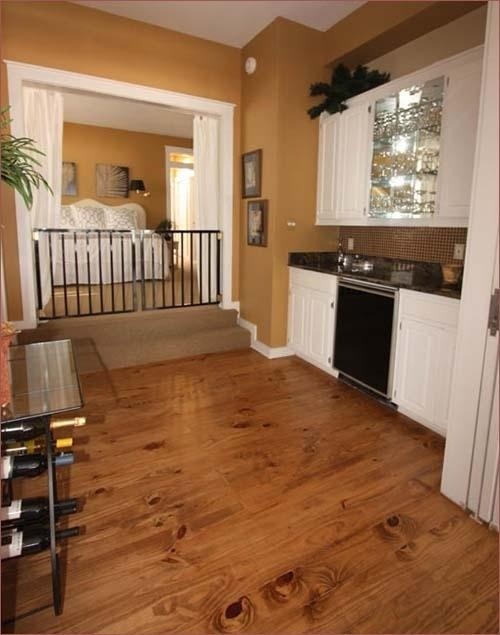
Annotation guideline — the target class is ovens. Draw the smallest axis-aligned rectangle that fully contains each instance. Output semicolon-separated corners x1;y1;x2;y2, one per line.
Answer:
331;277;398;400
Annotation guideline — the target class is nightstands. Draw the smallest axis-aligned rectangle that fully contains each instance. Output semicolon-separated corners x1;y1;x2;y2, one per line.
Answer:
165;240;180;270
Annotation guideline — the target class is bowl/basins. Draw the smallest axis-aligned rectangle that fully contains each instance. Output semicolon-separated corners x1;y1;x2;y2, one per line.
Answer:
441;264;462;283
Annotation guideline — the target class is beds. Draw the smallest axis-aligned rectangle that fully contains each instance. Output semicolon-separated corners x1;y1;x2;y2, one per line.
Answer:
47;195;172;289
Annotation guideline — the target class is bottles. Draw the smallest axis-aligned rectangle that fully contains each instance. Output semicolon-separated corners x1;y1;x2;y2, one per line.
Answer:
336;243;345;266
0;413;88;557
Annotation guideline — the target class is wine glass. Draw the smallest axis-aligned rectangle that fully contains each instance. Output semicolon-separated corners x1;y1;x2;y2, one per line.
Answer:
370;91;443;213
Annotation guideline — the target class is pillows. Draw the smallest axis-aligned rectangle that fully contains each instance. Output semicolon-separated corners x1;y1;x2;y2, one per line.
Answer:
103;205;140;230
71;204;106;228
59;205;76;229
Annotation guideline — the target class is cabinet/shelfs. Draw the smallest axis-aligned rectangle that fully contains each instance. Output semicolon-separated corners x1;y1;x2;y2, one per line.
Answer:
430;40;490;231
314;87;374;229
1;333;84;630
389;284;462;438
285;261;339;380
368;59;445;229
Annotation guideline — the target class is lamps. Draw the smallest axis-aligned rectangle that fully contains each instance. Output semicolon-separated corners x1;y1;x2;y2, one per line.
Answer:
128;178;145;197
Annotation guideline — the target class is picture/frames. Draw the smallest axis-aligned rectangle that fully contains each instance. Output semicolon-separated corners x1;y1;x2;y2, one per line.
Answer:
246;198;269;249
239;149;264;200
